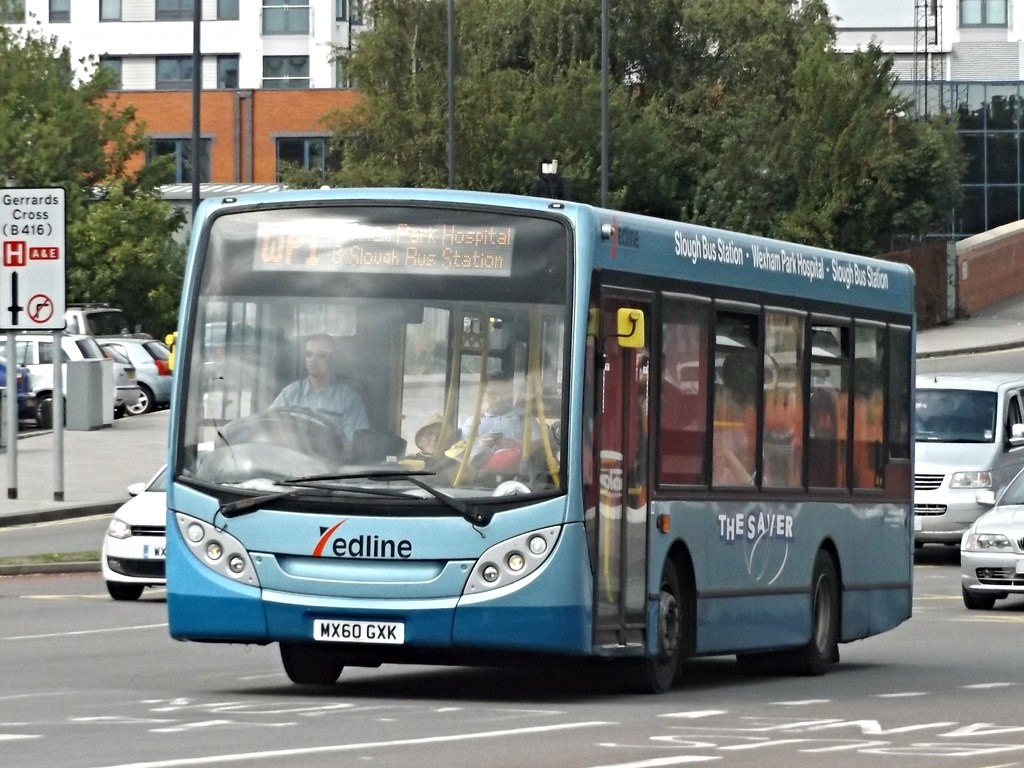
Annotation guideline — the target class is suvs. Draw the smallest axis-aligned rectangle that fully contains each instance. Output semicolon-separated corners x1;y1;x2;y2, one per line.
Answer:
60;302;132;339
0;332;109;428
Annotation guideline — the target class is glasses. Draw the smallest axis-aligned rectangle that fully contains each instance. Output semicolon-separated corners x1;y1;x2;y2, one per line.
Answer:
485;391;511;399
304;349;333;359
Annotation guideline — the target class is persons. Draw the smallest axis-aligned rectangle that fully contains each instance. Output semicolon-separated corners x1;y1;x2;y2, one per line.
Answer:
759;394;815;486
708;429;762;489
163;320;187;375
460;368;544;475
262;332;370;460
845;354;887;442
399;412;469;487
633;352;687;432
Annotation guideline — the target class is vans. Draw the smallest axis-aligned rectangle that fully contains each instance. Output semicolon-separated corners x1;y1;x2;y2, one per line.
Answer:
912;372;1024;551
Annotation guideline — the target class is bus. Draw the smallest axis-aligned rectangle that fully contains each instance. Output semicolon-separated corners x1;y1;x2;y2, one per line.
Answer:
165;187;919;690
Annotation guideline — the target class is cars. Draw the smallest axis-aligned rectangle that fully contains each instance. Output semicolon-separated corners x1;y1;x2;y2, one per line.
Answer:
202;319;262;390
93;339;140;421
1;356;32;432
99;331;177;415
101;464;171;604
959;468;1024;612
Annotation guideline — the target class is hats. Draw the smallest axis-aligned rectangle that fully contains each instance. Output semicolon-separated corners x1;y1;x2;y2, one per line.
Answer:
415;414;453;451
